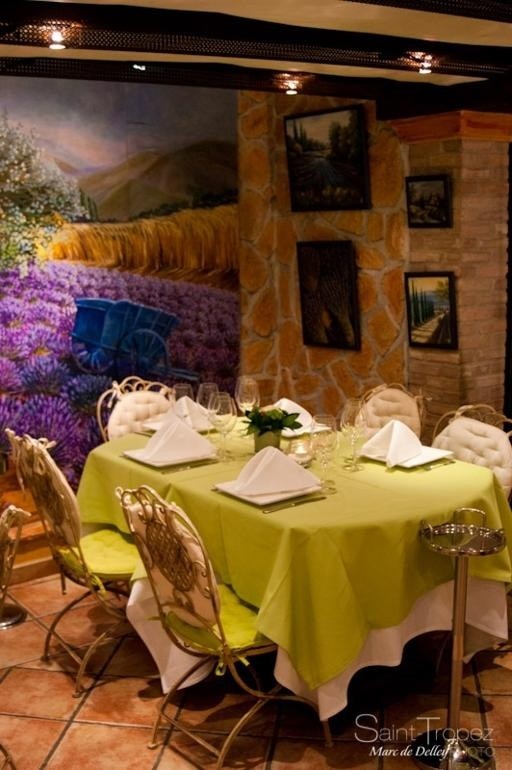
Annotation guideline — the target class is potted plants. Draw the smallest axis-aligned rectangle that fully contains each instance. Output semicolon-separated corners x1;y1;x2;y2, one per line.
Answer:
240;405;303;452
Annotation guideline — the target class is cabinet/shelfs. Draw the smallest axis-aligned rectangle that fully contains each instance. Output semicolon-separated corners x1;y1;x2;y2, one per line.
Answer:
406;506;506;770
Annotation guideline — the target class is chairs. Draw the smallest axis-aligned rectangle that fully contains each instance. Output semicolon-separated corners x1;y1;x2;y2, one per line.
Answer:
354;383;427;440
114;482;334;770
96;376;176;442
431;402;512;502
4;425;142;698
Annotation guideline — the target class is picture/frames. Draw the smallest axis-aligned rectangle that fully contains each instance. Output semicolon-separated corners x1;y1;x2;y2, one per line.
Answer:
283;104;373;212
405;174;453;229
296;239;360;351
403;271;458;351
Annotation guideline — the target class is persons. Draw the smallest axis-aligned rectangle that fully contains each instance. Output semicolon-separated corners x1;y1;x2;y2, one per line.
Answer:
284;110;363;207
408;187;448;225
301;246;355;345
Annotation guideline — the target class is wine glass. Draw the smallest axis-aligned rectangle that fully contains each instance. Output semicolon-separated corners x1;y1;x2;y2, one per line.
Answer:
309;396;368;497
172;375;259;462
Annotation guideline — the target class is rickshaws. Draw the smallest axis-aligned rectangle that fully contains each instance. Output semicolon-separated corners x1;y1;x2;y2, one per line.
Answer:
68;295;201;388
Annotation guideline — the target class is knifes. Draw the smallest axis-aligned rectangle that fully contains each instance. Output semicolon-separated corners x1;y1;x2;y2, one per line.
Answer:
162;462;212;474
264;497;326;514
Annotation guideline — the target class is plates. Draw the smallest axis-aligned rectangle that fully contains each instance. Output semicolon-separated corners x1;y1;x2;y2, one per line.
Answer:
363;443;454;468
214;482;321;506
282;422;331;438
143;421;216;435
125;449;215;466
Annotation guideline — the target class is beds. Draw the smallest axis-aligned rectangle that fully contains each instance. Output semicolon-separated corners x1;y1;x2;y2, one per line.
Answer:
75;414;511;722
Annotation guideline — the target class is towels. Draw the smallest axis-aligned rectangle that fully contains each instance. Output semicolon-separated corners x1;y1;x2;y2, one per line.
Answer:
139;416;219;463
159;393;216;432
259;394;315;435
359;418;423;468
234;445;321;496
